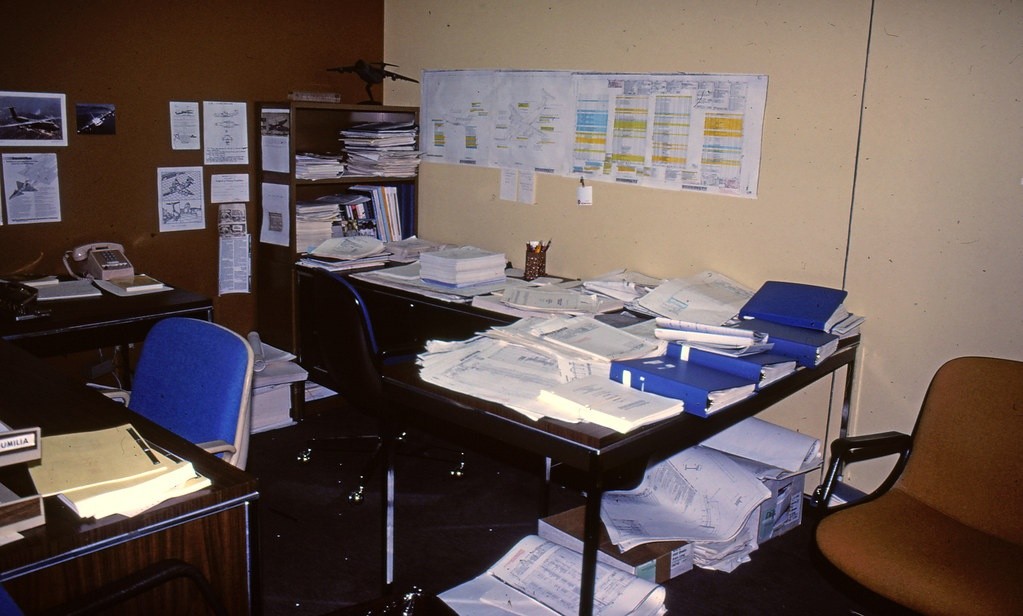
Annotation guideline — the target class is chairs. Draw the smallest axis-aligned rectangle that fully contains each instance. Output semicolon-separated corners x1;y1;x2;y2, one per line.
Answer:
296;264;472;506
809;352;1023;616
101;315;258;469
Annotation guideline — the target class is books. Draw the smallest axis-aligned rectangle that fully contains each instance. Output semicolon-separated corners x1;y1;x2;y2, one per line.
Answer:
248;120;865;616
19;274;174;300
27;423;212;520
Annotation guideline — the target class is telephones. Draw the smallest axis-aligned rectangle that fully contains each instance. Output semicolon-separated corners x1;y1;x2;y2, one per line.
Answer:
71;241;135;286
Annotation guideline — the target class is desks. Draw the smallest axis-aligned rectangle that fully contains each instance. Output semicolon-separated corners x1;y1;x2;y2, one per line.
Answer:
0;239;862;616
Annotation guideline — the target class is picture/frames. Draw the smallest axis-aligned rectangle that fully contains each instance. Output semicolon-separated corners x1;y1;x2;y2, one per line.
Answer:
0;91;69;147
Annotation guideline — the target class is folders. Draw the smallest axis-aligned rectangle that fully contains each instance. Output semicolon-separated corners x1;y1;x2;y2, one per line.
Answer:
728;319;840;368
666;339;797;390
737;279;853;333
609;355;758;420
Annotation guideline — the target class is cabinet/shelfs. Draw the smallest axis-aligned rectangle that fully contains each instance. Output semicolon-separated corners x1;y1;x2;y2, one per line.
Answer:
251;100;422;356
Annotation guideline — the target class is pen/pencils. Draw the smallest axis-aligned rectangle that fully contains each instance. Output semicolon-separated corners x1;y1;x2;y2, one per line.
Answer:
525;239;552;253
578;176;585;204
